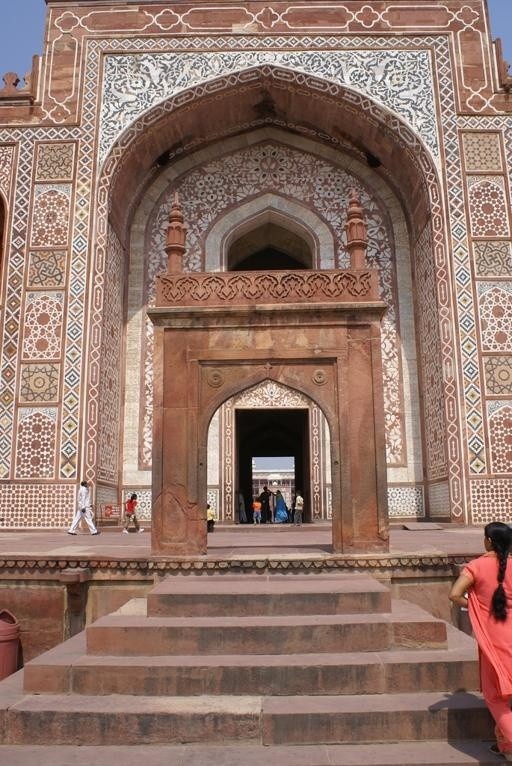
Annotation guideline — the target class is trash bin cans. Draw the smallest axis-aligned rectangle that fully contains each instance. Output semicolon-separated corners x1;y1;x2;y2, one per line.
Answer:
458;591;472;635
0;608;20;682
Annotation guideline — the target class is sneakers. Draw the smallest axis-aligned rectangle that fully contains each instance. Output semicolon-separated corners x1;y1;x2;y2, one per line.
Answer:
137;528;145;533
122;529;129;534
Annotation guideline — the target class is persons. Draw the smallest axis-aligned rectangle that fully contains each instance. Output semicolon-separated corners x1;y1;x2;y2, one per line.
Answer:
207;505;214;532
449;522;512;766
68;480;100;535
122;494;144;534
235;486;304;527
